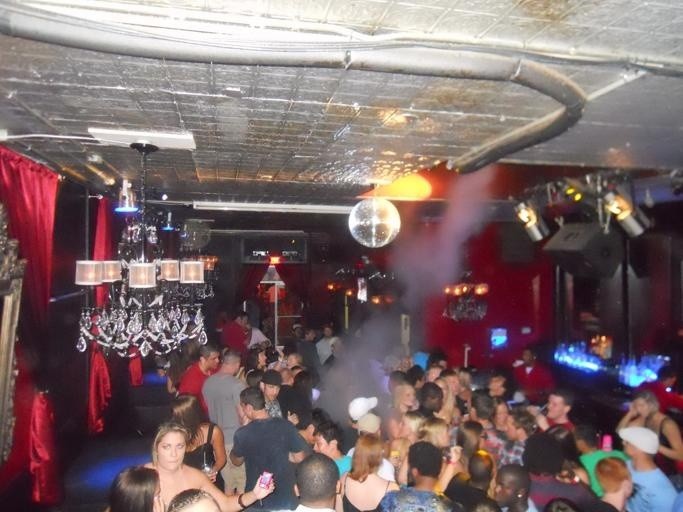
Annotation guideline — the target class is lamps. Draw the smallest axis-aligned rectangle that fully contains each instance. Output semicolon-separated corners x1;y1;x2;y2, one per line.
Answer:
322;254;400;306
71;129;212;357
513;170;652;276
115;172;184;238
347;194;401;249
437;228;490;323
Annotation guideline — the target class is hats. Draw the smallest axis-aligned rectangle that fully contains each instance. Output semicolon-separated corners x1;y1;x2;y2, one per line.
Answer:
357;413;381;436
618;426;660;456
260;370;282;386
347;396;378;421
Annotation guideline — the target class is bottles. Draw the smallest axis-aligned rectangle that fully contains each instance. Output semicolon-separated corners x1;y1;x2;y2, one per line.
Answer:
555;339;664;388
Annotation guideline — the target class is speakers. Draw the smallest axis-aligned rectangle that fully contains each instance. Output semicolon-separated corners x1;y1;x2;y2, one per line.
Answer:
542;222;624;279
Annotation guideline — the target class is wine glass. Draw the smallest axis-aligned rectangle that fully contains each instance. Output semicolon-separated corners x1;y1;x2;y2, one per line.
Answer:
202;451;218;482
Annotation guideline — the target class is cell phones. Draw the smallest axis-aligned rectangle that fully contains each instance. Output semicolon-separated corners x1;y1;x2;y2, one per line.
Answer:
259;471;274;490
